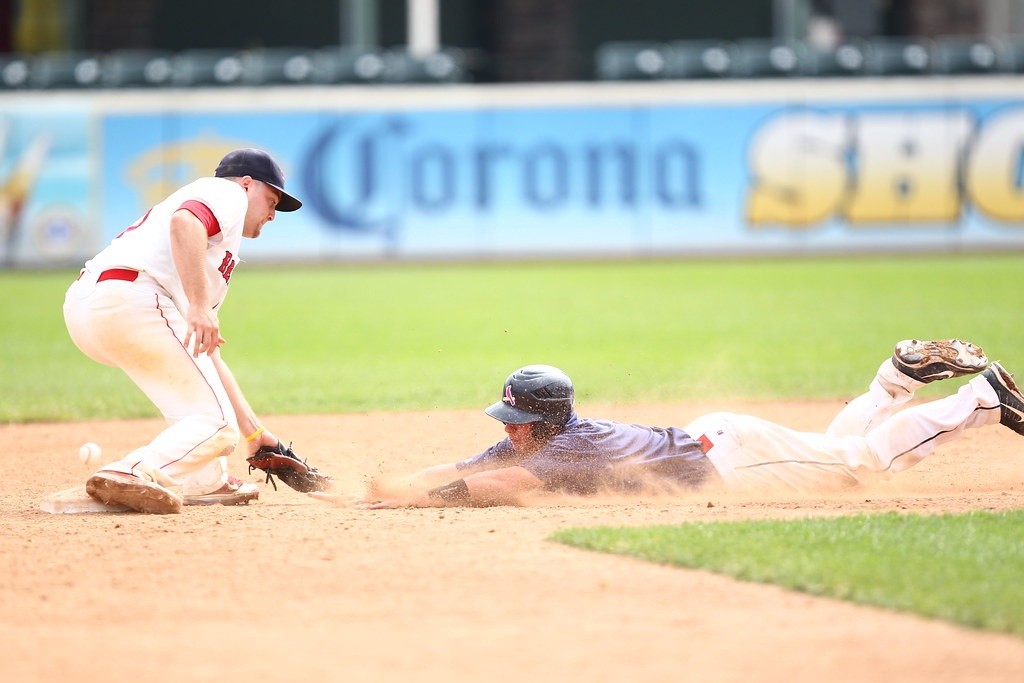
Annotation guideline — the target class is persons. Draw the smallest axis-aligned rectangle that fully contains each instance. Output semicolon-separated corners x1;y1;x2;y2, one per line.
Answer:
309;338;1024;508
60;148;329;515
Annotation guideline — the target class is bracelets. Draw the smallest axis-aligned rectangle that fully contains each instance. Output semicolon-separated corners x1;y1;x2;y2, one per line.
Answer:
243;426;265;444
429;478;470;507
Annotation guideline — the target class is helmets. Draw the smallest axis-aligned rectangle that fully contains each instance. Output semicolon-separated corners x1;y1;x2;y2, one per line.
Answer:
485;364;574;425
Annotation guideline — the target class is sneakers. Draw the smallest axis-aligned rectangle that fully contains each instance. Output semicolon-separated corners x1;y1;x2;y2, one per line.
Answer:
982;360;1024;436
86;470;182;514
892;338;988;385
182;475;259;505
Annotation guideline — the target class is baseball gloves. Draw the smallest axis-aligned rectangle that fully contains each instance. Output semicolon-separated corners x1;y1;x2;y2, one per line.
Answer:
244;440;330;494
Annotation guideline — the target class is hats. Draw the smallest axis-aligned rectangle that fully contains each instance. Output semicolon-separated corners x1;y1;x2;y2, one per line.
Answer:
215;148;302;212
